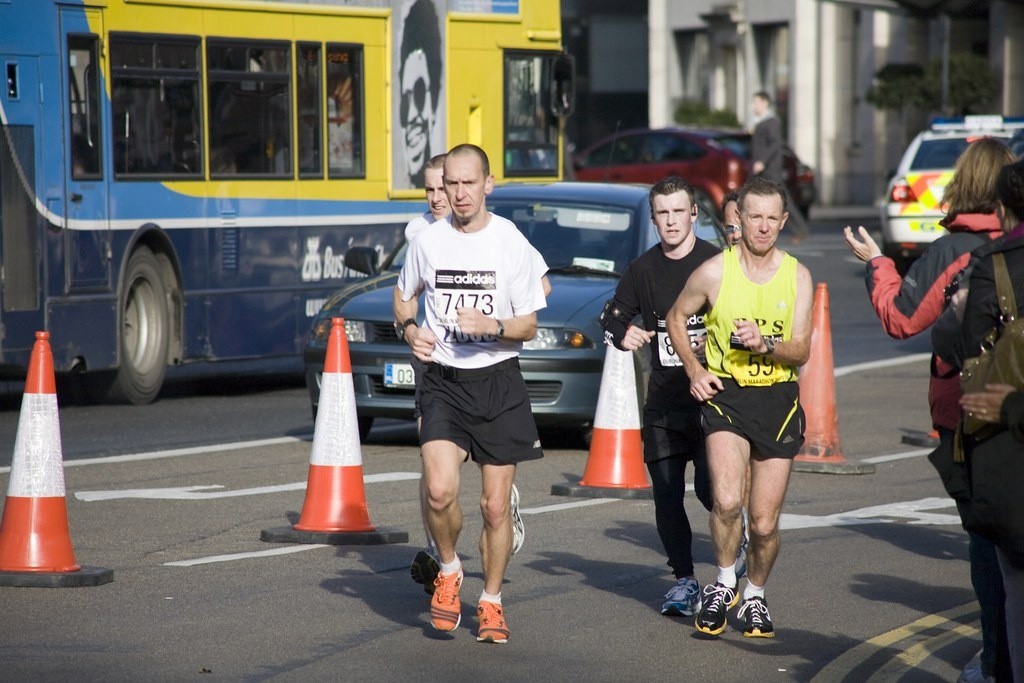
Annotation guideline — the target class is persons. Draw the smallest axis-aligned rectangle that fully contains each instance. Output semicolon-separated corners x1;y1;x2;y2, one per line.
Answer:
393;144;547;644
402;153;553;596
749;91;811;247
599;175;749;618
666;178;813;638
843;127;1024;683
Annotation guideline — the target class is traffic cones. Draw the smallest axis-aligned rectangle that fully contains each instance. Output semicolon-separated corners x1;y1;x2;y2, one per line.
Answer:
551;343;655;500
900;430;942;448
791;282;876;474
0;331;114;588
260;317;409;545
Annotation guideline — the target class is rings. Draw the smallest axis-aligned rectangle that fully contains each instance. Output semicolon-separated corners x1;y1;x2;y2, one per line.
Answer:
981;407;987;415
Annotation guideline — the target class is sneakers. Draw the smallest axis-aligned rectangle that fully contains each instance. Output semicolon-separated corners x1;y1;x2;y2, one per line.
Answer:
695;573;739;635
476;599;510;644
511;483;524;555
661;576;703;618
735;546;747;576
736;597;775;638
409;548;439;596
430;558;463;632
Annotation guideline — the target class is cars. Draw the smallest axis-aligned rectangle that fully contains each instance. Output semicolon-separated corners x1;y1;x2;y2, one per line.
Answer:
306;182;733;447
571;127;817;245
878;115;1024;278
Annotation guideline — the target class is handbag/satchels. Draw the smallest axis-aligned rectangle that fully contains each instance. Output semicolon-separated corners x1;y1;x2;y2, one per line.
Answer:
958;253;1023;433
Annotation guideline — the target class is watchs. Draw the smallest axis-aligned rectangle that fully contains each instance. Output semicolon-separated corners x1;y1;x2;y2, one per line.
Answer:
761;339;775;356
494;319;504;339
395;318;418;344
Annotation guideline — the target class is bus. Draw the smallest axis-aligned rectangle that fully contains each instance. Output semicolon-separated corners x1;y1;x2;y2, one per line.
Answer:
1;0;576;405
